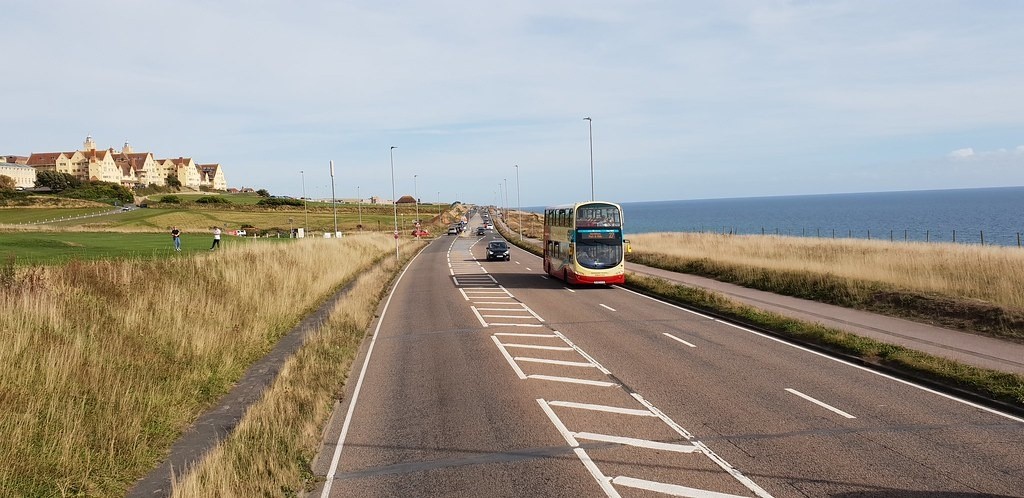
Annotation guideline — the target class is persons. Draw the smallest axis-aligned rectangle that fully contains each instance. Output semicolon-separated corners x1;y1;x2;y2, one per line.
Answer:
210;226;221;250
599;214;614;226
577;248;588;258
171;226;181;251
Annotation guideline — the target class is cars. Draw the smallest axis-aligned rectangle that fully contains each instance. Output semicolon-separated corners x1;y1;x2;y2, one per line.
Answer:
483;213;494;230
411;230;430;237
236;230;246;237
476;227;486;236
486;241;510;262
448;226;467;235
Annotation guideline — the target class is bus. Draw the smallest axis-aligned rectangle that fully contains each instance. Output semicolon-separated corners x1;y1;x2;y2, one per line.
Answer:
542;201;632;286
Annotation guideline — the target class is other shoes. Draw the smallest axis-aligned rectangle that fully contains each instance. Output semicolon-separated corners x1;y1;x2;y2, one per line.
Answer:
174;249;181;252
209;247;213;250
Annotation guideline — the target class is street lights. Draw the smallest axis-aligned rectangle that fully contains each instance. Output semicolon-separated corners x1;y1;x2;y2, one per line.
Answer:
415;175;419;241
583;117;594;201
504;179;510;233
391;146;399;259
301;171;308;237
515;165;522;241
